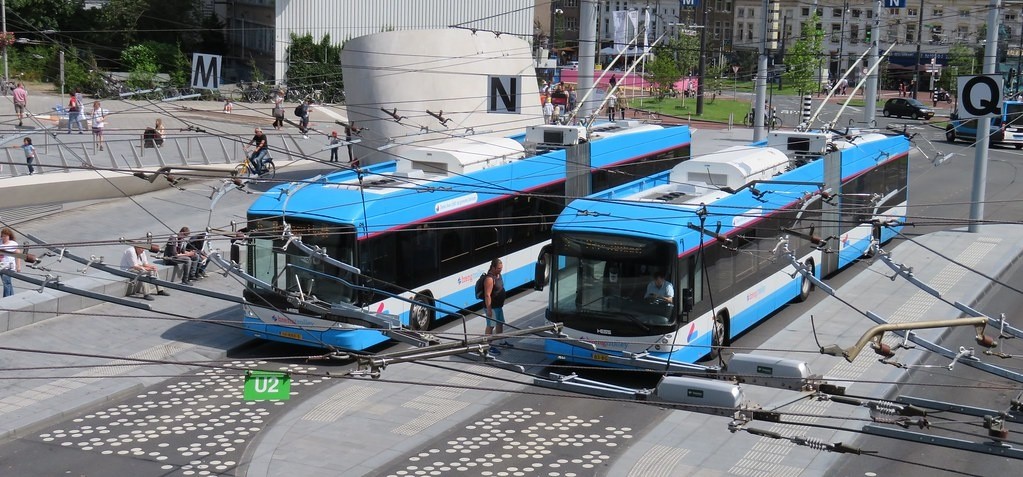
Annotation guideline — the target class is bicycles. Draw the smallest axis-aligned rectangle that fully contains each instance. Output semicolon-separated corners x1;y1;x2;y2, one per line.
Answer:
743;107;783;130
97;82;194;101
231;80;345;104
233;149;275;186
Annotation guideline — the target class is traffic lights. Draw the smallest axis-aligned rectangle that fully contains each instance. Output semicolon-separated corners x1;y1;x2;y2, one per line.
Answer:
866;30;871;42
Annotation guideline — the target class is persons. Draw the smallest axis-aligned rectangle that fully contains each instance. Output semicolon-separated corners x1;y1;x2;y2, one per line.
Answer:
155;119;166;143
0;228;20;297
606;73;628;122
824;76;848;95
1003;93;1022;102
120;227;209;301
68;91;108;151
223;99;232;114
752;96;770;125
330;124;357;163
272;90;286;131
12;137;36;175
483;258;515;356
538;80;575;125
898;80;913;97
939;85;945;97
242;127;268;178
13;82;27;126
299;98;315;139
644;271;674;304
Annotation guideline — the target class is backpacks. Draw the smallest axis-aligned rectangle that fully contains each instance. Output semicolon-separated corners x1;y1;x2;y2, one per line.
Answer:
475;273;494;299
294;105;304;117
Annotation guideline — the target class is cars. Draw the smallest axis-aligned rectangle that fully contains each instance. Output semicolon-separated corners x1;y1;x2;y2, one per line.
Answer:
883;97;935;120
930;87;950;101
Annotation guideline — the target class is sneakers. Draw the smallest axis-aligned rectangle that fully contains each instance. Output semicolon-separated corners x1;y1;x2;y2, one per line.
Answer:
489;346;501;355
499;341;513;347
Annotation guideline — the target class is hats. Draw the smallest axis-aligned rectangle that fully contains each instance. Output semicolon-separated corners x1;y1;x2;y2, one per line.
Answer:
332;131;337;136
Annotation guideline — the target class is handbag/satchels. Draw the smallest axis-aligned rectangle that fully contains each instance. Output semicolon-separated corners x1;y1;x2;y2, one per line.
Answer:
78;108;86;121
272;108;284;117
76;98;83;112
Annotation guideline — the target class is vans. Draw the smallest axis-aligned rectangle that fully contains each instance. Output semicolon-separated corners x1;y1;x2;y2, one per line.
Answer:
945;100;1023;150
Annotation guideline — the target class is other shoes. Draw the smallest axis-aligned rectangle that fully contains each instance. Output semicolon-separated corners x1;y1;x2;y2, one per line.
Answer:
19;121;23;126
254;170;260;173
158;290;170;296
144;294;154;301
100;145;104;151
303;135;308;139
79;132;83;134
31;169;36;174
182;273;209;285
27;172;32;175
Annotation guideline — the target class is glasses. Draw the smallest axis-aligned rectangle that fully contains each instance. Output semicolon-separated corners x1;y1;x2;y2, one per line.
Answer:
255;131;260;133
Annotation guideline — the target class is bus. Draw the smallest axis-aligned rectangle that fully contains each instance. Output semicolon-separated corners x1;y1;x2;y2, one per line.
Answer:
534;44;910;376
230;31;692;351
